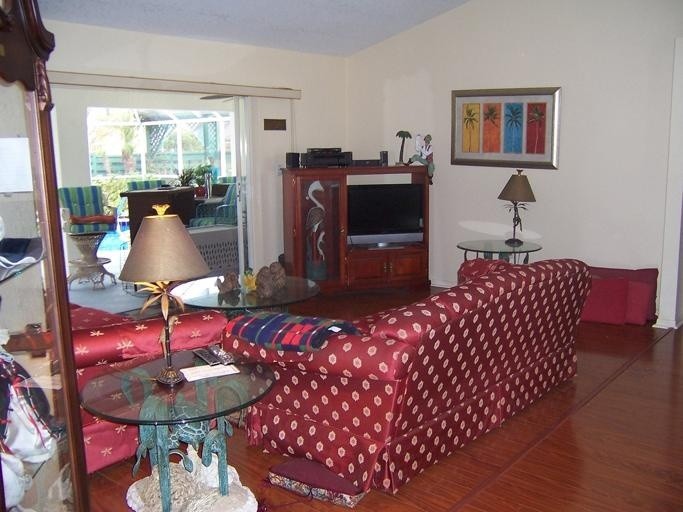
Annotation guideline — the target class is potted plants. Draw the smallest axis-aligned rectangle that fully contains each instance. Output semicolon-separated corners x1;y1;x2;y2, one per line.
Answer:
193;163;214;200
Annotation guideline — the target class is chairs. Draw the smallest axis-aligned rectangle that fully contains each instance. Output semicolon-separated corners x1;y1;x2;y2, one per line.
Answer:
54;171;237;238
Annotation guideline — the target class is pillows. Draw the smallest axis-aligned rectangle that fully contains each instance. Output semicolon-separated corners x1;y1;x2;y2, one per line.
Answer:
578;275;630;327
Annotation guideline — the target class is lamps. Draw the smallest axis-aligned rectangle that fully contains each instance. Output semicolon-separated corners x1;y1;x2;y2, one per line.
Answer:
495;169;536;246
112;201;213;394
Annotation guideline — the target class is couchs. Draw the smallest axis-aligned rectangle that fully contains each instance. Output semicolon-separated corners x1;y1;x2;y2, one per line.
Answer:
40;283;231;479
220;254;594;508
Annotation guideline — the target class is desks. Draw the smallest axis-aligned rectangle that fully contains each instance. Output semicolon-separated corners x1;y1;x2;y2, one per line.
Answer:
166;264;325;431
77;346;275;511
455;239;543;265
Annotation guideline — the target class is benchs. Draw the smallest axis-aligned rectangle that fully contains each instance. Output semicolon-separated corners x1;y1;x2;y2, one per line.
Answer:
578;265;659;326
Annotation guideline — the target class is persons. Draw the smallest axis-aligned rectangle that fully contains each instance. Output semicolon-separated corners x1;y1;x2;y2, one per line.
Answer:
394;130;434;185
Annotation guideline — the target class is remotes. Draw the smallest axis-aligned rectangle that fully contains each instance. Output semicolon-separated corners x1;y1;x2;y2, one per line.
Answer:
192;347;221;366
207;342;235;366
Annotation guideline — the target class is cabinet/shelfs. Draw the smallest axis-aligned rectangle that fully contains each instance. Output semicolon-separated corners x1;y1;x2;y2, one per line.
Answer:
0;0;93;511
342;162;430;293
276;163;346;300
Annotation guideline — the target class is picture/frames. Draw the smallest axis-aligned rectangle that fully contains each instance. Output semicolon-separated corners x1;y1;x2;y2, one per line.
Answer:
448;86;562;170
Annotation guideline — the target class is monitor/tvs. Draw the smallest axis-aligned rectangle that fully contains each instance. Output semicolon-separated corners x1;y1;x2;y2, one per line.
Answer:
347;184;423;251
